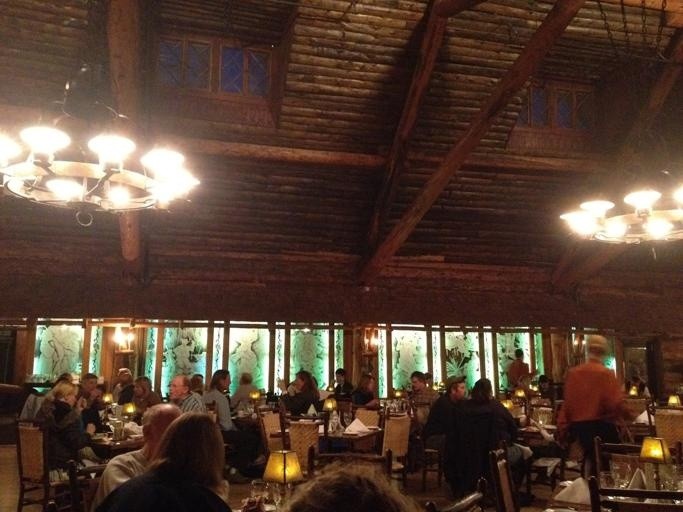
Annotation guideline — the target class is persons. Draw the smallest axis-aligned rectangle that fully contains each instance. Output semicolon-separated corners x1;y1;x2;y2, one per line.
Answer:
33;335;651;511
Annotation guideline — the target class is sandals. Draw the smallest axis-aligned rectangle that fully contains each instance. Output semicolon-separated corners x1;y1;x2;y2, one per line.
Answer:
251;454;266;467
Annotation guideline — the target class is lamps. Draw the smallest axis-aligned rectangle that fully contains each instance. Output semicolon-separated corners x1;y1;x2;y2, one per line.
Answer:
559;0;683;246
0;19;203;226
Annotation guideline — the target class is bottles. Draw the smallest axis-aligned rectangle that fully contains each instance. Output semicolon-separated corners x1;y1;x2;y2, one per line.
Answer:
112;416;127;439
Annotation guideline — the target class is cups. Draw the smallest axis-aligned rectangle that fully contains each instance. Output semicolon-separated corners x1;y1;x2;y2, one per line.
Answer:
538;414;547;424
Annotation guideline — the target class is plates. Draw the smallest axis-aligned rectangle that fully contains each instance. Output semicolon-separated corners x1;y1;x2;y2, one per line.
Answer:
89;432;143;442
520;424;558;434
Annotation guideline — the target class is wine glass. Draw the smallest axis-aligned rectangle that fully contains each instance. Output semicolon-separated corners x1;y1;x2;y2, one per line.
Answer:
381;398;409;414
241;400;276;419
317;411;353;433
248;479;292;511
598;461;683;511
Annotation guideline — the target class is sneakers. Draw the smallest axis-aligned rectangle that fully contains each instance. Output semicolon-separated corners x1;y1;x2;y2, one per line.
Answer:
225;469;250;484
